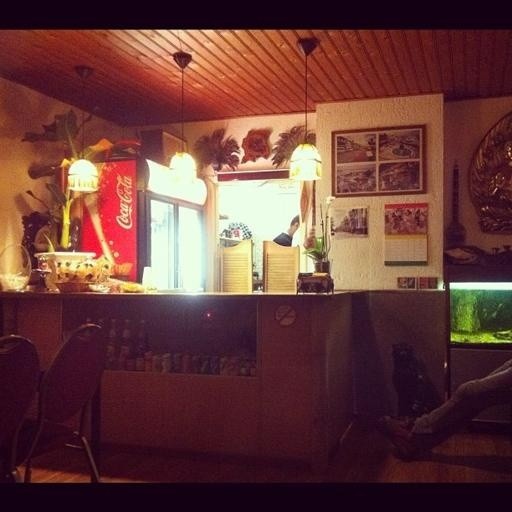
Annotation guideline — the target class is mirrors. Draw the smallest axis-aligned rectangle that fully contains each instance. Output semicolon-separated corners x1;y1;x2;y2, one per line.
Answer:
17;212;83;284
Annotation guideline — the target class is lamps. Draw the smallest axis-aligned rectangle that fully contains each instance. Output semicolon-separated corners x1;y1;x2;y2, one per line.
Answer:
68;65;100;193
169;51;196;190
286;36;322;182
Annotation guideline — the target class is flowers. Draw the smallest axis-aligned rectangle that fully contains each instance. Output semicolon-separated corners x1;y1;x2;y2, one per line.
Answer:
302;195;336;262
40;137;117;253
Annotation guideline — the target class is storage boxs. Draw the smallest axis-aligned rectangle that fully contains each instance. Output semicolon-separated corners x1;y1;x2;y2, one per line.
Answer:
141;130;189;169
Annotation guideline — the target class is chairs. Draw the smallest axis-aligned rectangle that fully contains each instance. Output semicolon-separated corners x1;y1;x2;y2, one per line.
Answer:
29;324;107;483
1;336;41;483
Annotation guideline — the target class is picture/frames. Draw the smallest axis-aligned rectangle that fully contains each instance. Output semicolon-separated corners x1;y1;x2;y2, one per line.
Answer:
330;124;428;197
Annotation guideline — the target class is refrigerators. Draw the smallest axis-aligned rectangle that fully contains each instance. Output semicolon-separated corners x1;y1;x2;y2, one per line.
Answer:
78;156;208;292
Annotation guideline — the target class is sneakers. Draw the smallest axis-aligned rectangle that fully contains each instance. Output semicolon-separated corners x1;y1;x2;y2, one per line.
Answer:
376;415;426;462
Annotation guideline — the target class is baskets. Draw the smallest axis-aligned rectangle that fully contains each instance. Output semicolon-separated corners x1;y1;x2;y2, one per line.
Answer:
0;244;32;292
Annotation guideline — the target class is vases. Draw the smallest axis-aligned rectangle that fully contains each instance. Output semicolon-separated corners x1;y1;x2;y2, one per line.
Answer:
33;251;97;293
313;263;331;273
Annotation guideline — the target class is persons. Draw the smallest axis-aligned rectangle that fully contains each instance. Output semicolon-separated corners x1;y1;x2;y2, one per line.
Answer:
274;215;300;246
376;358;512;459
